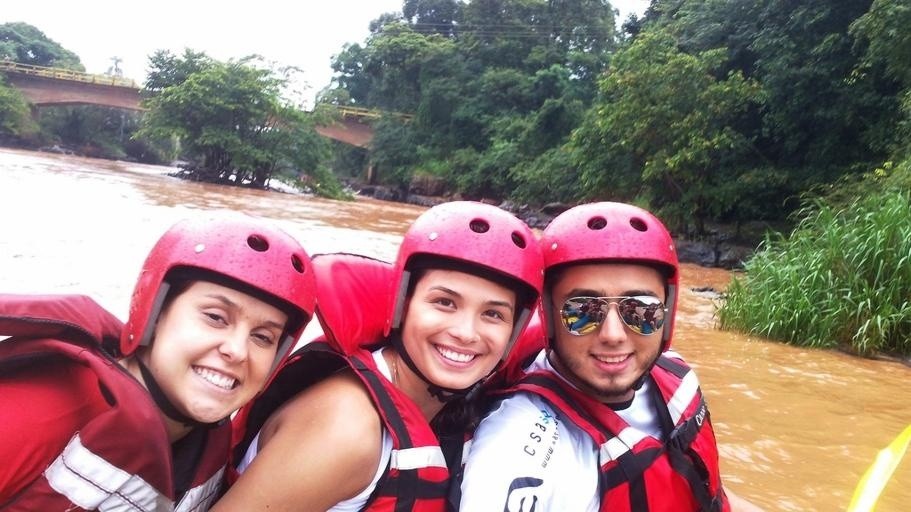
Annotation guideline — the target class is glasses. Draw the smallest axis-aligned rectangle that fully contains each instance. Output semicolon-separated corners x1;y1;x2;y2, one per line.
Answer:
559;296;668;336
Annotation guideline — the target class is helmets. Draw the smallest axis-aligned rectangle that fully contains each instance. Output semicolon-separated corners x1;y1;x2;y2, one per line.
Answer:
540;200;678;353
120;209;317;396
384;201;540;372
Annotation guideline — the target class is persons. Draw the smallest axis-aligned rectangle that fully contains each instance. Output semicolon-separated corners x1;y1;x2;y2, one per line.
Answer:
207;200;545;512
1;209;316;512
456;200;767;512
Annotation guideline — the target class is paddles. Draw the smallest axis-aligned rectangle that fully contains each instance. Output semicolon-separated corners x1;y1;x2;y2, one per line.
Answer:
828;426;910;512
